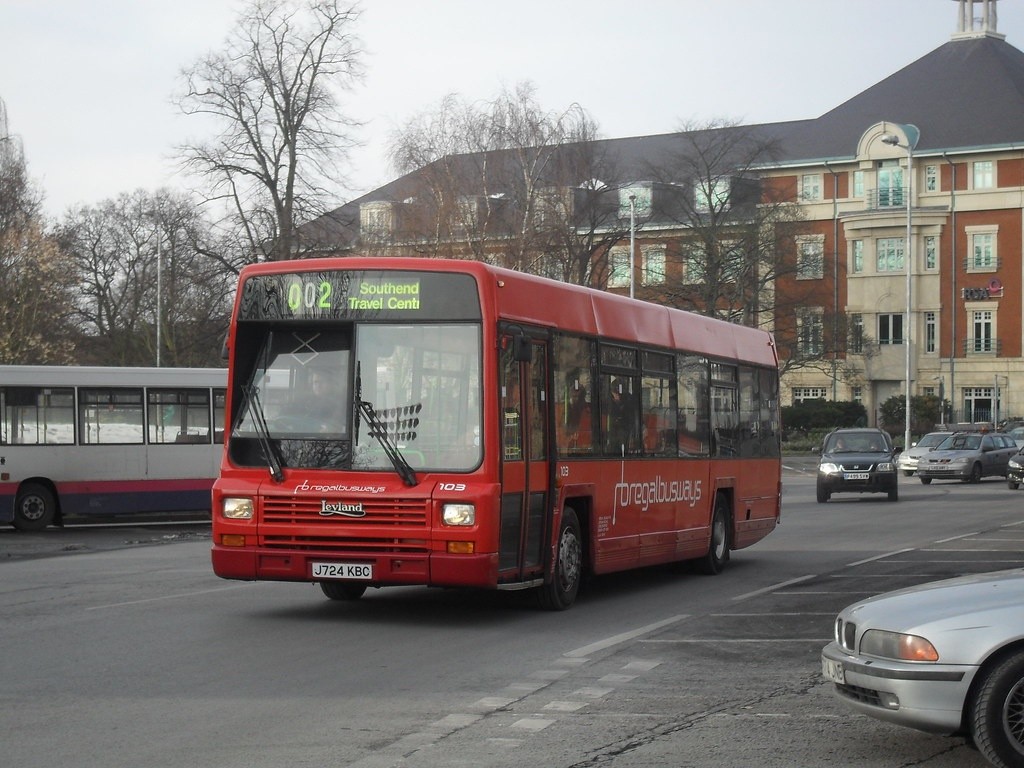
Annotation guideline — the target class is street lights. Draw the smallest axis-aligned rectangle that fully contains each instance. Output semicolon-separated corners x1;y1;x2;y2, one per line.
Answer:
881;132;915;450
145;210;163;368
628;194;637;299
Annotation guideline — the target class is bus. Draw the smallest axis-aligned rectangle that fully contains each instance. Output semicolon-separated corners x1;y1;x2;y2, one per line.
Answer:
212;255;784;613
0;365;295;534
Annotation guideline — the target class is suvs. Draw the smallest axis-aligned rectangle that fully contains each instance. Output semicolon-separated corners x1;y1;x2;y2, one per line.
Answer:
811;426;904;502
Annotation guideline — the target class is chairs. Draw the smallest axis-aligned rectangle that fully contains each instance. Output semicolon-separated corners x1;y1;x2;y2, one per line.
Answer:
206;429;224;443
542;401;695;454
175;430;199;443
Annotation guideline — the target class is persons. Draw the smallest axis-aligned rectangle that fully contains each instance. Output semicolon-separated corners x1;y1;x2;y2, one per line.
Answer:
869;439;881;451
611;377;628;401
562;383;586;431
829;437;852;453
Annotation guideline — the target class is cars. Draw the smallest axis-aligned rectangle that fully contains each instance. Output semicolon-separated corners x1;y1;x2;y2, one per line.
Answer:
1006;446;1024;490
917;432;1020;485
1002;420;1024;450
898;431;968;476
821;568;1023;768
